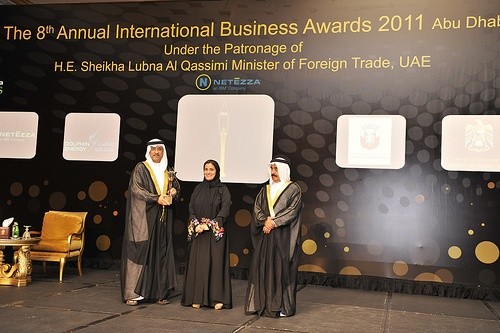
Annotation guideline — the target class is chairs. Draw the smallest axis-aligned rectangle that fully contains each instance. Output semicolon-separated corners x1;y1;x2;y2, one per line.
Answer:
14;211;88;283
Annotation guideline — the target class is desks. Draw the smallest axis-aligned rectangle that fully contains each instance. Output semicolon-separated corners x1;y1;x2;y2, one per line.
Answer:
0;237;41;287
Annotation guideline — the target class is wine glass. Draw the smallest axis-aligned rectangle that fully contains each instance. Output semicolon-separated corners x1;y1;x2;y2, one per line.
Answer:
22;225;31;239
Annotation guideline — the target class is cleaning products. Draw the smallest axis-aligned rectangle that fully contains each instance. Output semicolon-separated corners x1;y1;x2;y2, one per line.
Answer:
22;225;31;240
12;222;19;239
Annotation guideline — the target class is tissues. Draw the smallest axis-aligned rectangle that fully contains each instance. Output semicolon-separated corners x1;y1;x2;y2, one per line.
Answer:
0;217;14;239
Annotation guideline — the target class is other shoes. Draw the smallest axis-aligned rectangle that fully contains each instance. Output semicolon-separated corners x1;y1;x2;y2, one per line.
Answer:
193;303;200;309
158;298;169;305
215;303;223;310
127;300;137;305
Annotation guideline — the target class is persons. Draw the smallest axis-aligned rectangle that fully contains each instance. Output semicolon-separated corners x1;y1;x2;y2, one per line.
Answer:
120;138;181;306
180;159;233;310
244;153;304;319
